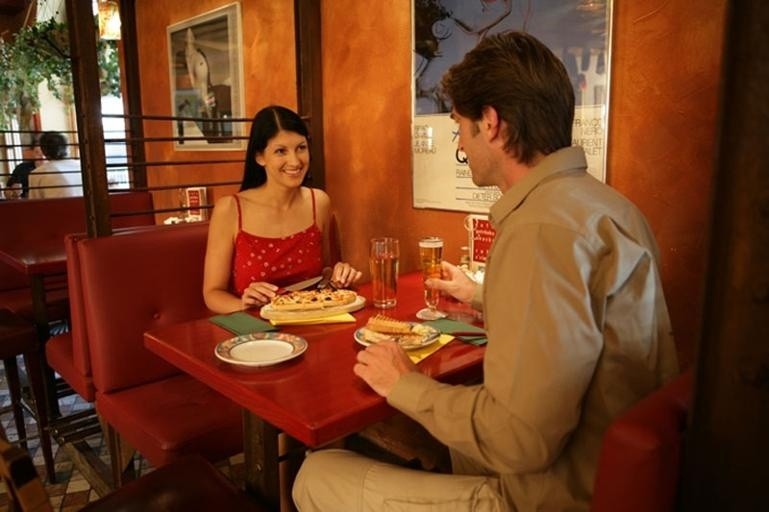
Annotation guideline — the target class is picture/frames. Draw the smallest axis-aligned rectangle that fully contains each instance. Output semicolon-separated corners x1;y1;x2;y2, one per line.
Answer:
167;2;247;151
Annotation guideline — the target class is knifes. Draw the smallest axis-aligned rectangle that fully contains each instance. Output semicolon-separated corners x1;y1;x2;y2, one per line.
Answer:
276;275;324;295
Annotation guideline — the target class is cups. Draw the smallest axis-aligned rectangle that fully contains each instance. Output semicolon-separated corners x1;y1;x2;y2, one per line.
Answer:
369;238;399;309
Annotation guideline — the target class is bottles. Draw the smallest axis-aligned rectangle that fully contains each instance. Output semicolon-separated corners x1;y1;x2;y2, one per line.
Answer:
458;246;469;273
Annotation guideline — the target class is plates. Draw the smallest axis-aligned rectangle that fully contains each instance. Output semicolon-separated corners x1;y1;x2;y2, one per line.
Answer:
214;331;309;370
352;321;441;355
259;295;367;320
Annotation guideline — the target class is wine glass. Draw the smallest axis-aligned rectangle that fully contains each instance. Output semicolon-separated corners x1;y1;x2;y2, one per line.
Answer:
4;184;23;200
415;236;449;320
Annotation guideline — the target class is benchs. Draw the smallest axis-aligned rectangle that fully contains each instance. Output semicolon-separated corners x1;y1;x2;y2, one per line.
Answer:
2;192;699;512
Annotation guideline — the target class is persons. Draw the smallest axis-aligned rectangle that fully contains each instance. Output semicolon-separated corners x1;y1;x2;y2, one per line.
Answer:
4;131;83;200
204;104;363;315
292;29;677;511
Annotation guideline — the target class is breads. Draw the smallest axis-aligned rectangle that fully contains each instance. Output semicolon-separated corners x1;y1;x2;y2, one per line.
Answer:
364;314;439;346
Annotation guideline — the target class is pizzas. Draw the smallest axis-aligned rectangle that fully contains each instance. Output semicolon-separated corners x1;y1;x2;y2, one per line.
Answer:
271;288;357;312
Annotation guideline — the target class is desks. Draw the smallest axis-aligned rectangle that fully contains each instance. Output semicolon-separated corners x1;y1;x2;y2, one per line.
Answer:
3;239;73;477
143;271;485;511
